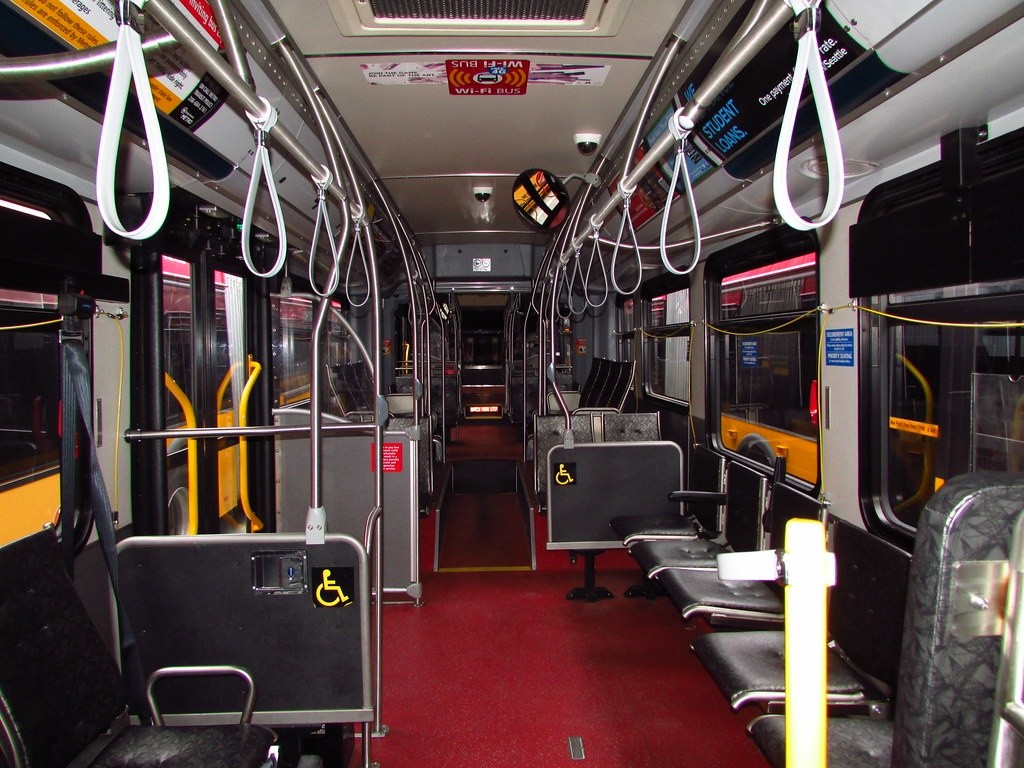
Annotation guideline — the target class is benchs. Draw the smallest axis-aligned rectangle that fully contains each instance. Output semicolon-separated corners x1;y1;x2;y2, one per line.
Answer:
2;357;1024;768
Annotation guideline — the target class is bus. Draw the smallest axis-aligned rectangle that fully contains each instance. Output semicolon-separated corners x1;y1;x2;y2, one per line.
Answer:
0;198;346;550
647;251;1024;530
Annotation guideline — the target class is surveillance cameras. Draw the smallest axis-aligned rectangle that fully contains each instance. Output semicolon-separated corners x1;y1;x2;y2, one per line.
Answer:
574;133;601;156
471;186;493;202
